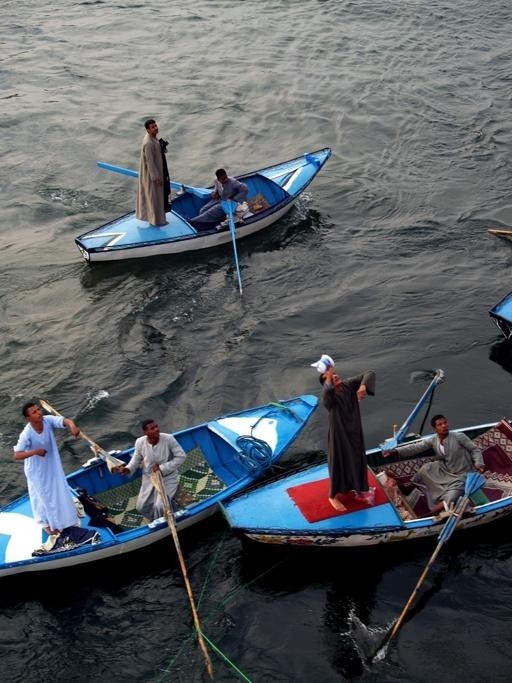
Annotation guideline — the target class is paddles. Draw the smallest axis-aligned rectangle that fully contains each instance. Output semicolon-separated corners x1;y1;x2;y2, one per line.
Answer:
489;229;511;238
374;472;486;665
40;400;125;473
97;162;214;198
379;369;444;457
222;200;242;296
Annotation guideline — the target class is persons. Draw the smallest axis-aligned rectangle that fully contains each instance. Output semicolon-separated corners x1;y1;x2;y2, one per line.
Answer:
118;419;187;522
13;401;81;536
382;415;485;518
188;168;248;223
135;118;172;227
319;364;376;512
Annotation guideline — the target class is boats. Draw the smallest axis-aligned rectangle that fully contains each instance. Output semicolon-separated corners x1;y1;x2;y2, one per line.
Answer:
487;289;512;341
74;147;332;263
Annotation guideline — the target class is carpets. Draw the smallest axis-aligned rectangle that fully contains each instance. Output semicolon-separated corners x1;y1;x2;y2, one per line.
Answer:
87;445;228;530
287;466;392;524
246;192;271;214
376;419;512;521
